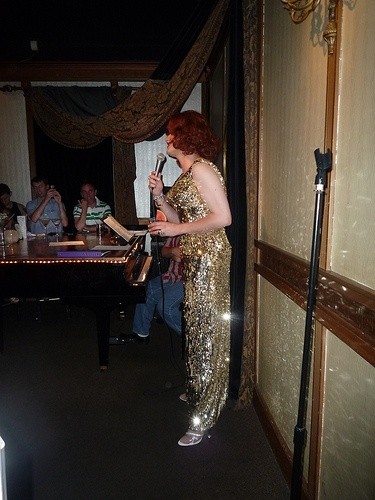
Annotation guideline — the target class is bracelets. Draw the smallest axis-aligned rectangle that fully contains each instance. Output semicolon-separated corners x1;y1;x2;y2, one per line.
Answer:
153;194;164;201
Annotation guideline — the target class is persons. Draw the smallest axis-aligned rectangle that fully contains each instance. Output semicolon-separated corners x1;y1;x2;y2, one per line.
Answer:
121;236;183;343
73;180;112;232
25;176;69;234
146;111;234;446
0;183;26;232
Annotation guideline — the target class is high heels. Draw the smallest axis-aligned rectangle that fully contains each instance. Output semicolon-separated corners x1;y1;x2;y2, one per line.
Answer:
179;422;212;447
179;393;188;402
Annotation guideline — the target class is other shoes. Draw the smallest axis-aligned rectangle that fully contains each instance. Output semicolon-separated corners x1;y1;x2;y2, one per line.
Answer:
4;297;19;303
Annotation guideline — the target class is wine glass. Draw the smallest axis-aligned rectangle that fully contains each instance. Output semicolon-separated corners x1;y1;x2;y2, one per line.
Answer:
39;218;51;240
51;219;62;238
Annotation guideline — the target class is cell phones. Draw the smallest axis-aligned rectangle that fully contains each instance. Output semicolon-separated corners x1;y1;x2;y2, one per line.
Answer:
51;185;54;189
82;228;88;232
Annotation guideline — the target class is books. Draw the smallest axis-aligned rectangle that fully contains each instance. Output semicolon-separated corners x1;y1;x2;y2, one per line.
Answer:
56;250;110;258
102;215;133;242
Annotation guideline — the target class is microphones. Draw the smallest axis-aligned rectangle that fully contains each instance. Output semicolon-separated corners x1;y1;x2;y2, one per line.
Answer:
152;153;165;190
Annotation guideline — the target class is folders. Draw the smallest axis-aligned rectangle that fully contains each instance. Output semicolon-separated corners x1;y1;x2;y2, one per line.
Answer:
57;250;109;258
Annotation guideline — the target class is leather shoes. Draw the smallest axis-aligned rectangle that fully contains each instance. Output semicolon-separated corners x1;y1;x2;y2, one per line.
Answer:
115;333;150;345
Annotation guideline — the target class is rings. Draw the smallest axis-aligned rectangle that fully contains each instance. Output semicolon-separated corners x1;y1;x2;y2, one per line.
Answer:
158;230;162;235
